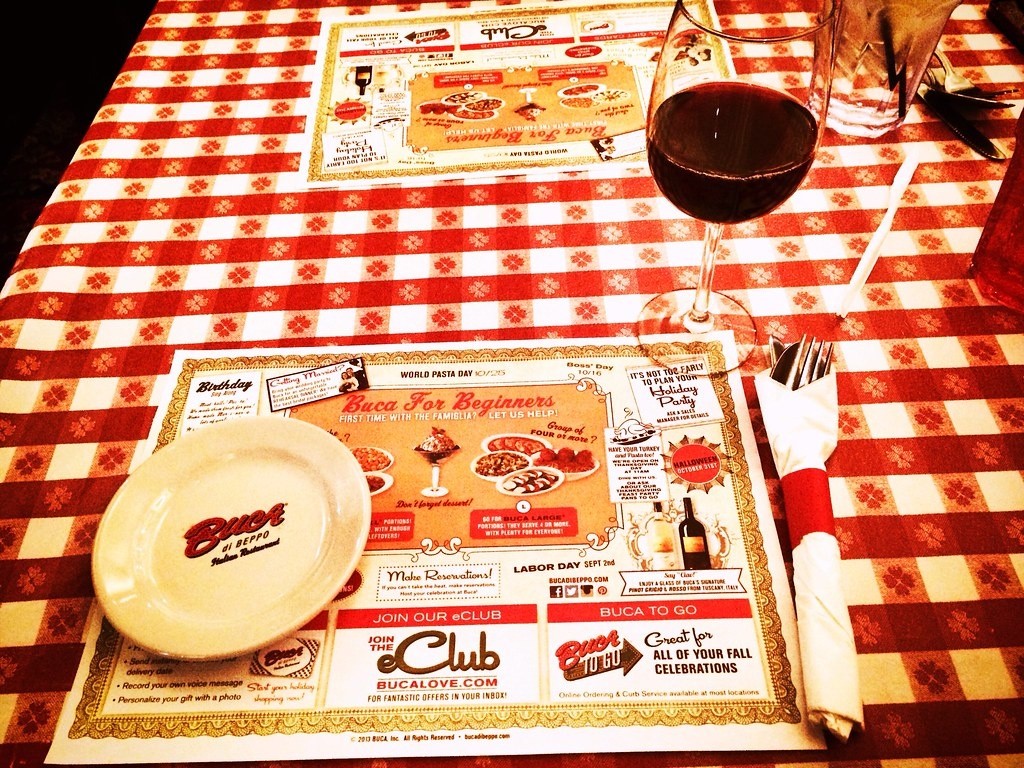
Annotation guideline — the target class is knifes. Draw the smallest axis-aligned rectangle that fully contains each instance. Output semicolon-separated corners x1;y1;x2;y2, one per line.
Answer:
769;334;786;368
917;84;1006;161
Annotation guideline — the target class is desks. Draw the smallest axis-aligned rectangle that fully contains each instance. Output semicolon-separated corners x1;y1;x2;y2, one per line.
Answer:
0;0;1024;768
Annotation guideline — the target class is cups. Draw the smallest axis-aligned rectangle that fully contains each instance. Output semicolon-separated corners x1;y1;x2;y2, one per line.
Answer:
810;0;962;138
969;108;1024;316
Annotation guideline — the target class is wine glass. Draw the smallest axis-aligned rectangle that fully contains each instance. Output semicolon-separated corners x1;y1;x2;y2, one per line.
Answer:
636;2;836;377
415;447;461;498
514;88;546;113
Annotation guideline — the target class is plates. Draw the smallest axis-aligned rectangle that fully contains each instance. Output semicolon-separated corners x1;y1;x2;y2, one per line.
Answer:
442;93;488;105
351;448;394;473
463;99;504;112
482;433;553;458
593;90;629;102
560;99;593;110
558;83;606;98
91;419;372;663
365;472;394;496
470;451;532;481
416;101;448;114
448;110;499;121
496;467;565;496
531;450;600;481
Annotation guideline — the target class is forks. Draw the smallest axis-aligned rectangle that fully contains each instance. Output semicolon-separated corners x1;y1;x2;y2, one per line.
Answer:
934;47;1018;98
785;335;834;389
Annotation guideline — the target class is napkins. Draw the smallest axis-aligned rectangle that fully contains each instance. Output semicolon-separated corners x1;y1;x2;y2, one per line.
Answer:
751;367;868;743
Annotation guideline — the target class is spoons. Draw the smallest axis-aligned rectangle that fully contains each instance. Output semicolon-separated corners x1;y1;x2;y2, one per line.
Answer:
926;67;1016;108
770;342;829;390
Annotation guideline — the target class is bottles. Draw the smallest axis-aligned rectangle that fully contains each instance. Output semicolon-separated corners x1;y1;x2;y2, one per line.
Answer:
375;68;388;92
679;497;712;569
648;502;677;571
355;67;372;95
